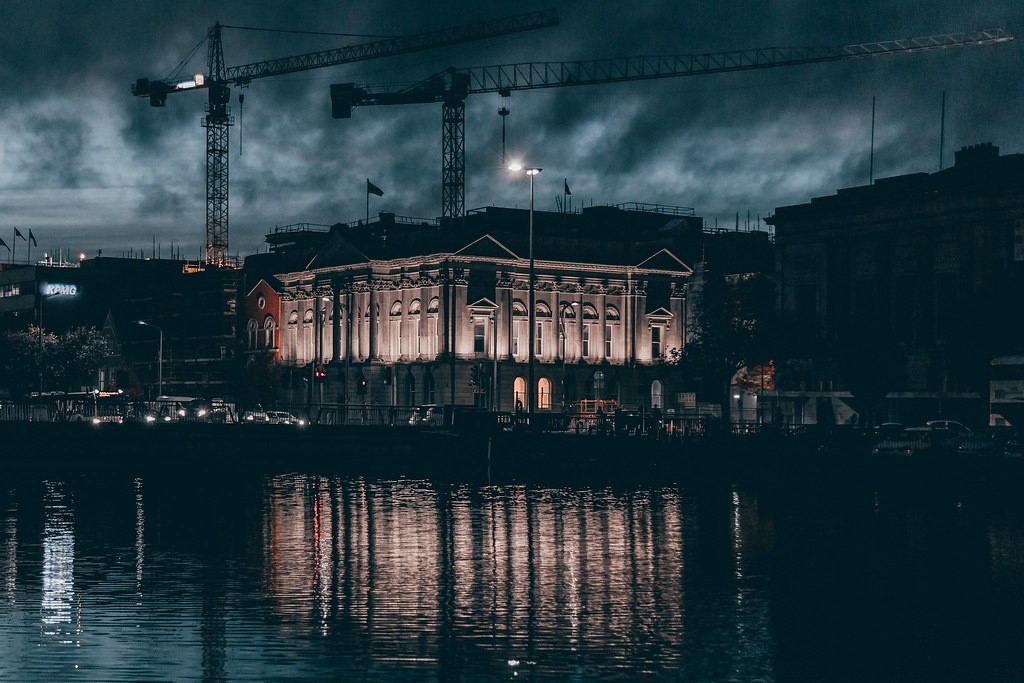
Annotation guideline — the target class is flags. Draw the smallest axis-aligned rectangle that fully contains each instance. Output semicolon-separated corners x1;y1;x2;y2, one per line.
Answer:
16;229;26;241
30;231;38;247
369;182;384;196
0;237;5;245
566;182;571;195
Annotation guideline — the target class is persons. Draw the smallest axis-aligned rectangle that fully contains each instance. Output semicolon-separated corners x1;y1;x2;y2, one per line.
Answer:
387;406;395;426
361;401;368;425
596;406;606;421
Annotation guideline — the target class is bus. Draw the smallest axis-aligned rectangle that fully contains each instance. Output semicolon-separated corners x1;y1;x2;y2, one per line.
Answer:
24;390;123;424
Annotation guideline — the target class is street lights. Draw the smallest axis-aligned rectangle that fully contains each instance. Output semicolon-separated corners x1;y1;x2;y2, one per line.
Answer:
507;162;543;423
138;319;164;397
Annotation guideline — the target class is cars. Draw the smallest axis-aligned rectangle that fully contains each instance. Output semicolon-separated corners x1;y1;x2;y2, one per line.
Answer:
408;404;445;427
126;401;304;426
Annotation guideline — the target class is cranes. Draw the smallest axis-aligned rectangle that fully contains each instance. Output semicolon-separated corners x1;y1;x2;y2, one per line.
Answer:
130;5;562;264
329;25;1016;221
